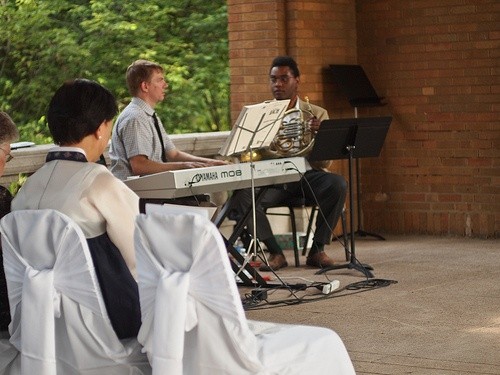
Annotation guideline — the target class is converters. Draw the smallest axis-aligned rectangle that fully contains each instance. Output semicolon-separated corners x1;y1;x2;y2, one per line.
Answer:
251;289;267;300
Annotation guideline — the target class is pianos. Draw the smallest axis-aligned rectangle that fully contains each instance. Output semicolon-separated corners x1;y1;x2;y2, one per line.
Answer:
123;157;306;301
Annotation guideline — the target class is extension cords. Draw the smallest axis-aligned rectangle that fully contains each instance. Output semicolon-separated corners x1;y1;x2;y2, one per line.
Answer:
323;280;340;295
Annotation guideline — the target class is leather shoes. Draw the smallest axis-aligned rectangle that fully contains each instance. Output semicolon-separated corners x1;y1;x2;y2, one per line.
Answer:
259;252;288;271
306;251;337;268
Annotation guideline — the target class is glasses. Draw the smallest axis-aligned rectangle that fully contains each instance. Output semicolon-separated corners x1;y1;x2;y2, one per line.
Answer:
270;74;295;84
0;147;13;163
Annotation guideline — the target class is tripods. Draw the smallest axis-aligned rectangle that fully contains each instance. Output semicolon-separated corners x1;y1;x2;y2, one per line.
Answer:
306;63;391;280
218;98;301;302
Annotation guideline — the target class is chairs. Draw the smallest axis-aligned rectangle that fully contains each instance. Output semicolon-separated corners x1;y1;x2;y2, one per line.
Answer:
0;209;152;375
265;205;350;267
132;209;358;375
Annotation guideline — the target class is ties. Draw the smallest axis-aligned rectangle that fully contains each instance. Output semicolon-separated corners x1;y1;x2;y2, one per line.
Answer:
152;112;167;162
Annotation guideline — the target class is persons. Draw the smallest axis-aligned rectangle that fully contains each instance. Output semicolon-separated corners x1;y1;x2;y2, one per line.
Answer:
0;110;21;332
106;59;229;238
230;56;347;271
10;77;218;343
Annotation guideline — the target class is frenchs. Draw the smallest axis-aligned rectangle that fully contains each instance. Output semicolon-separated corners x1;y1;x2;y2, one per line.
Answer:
241;96;317;163
